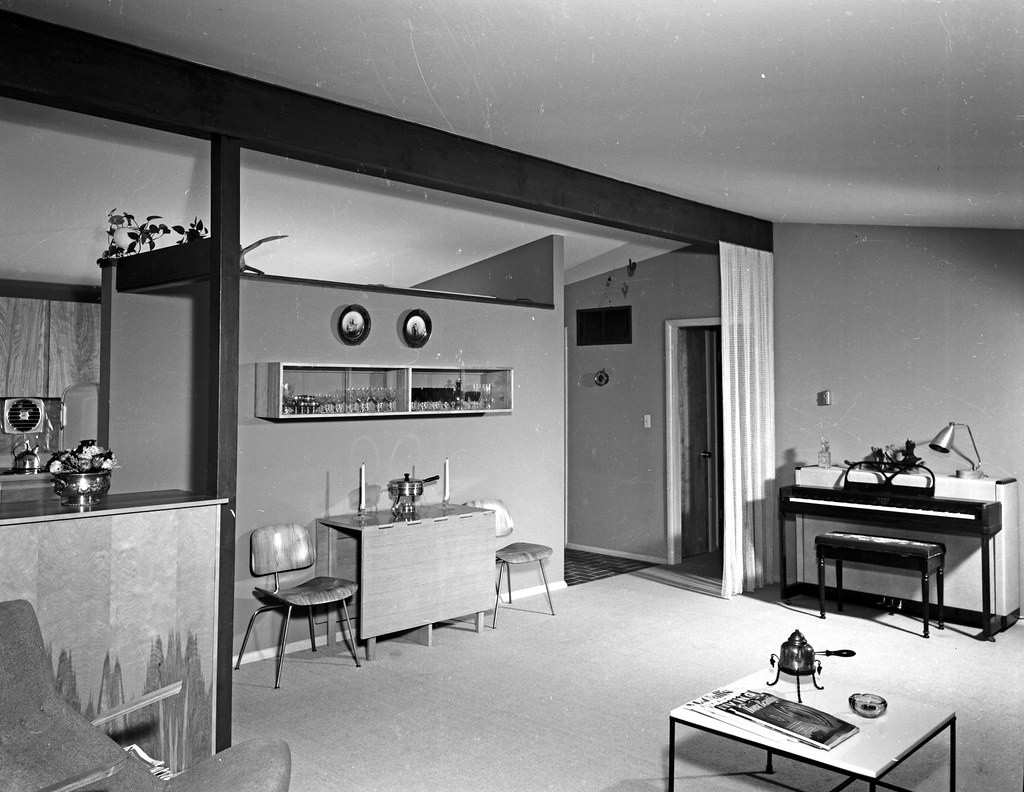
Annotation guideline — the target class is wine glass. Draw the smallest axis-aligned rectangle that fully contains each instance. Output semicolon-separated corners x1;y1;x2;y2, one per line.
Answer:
283;383;345;415
464;384;491;410
347;386;398;413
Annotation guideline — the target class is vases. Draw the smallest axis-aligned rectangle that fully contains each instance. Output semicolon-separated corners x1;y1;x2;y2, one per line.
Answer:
53;471;111;507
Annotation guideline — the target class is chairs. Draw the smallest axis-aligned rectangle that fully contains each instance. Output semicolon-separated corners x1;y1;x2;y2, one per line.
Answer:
0;601;292;792
237;524;360;689
467;499;555;629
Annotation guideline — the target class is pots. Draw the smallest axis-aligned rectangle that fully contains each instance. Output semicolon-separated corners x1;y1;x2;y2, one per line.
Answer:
388;473;440;496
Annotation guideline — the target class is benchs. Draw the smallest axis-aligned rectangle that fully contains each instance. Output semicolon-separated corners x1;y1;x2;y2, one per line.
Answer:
814;532;947;639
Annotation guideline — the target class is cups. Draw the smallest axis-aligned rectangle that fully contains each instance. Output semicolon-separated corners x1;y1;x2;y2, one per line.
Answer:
411;387;461;412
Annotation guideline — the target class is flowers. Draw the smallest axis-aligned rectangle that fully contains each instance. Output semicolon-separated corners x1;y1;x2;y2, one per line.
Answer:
47;437;119;478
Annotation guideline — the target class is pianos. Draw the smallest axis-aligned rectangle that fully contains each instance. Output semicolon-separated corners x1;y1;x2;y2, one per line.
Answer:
777;463;1024;643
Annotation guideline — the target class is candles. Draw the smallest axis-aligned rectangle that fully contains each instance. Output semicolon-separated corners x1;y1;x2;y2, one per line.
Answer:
360;461;366;510
444;457;449;498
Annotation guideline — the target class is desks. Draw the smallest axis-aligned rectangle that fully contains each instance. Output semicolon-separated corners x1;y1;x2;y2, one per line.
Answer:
669;669;957;792
314;506;497;660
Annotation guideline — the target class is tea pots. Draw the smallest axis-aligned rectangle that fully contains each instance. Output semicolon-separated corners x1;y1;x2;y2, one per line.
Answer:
778;629;856;674
11;442;42;469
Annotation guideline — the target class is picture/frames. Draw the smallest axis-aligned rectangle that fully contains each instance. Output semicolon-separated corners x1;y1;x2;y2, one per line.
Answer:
336;303;372;346
401;308;432;348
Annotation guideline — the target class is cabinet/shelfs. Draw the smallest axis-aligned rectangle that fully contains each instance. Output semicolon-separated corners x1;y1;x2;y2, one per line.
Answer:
265;361;514;418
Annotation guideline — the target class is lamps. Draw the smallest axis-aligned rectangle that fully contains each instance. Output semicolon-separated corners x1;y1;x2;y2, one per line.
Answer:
929;419;982;480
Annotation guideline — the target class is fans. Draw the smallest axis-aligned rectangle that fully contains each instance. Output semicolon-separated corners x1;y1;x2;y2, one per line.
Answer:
3;397;45;435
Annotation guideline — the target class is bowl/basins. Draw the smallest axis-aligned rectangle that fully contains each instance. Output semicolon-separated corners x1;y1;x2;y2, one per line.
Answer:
849;693;888;719
